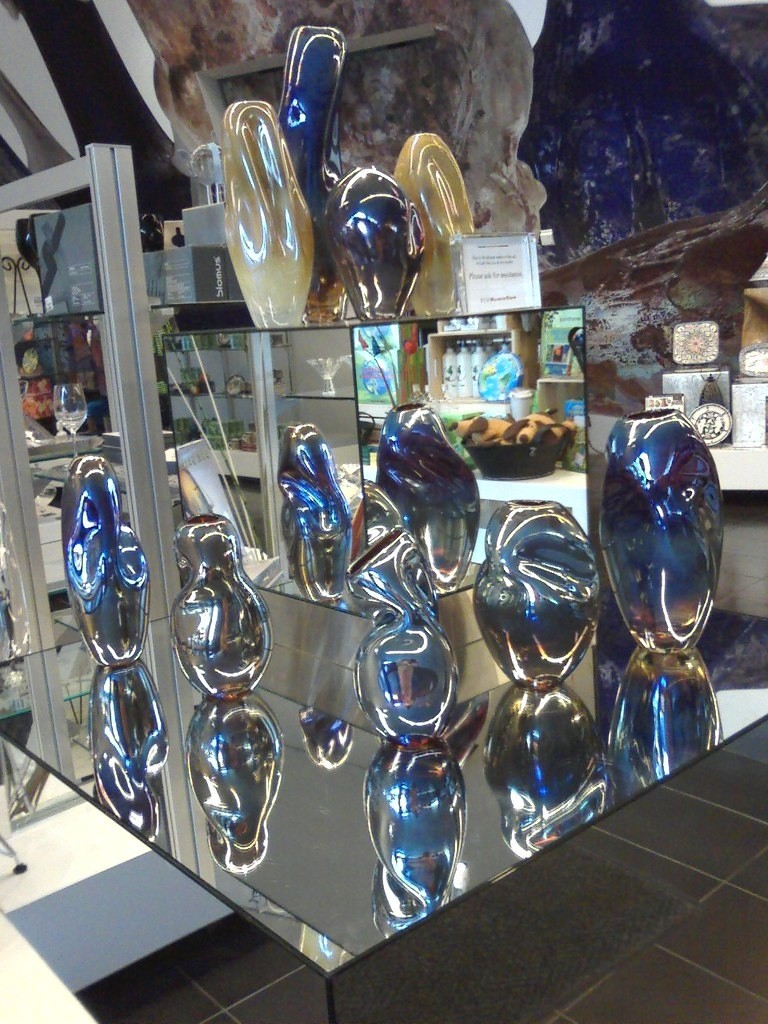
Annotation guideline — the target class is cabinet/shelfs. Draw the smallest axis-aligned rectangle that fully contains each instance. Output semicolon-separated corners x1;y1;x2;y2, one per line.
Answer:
161;303;598;754
0;144;194;873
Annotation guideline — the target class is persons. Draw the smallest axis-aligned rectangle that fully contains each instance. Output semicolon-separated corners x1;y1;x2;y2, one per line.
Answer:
82;330;109;437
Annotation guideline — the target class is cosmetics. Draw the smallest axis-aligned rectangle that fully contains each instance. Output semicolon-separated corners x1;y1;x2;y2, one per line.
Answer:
439;337;515;400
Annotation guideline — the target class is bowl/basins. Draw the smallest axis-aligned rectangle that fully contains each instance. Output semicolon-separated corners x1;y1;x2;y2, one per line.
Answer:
462;425;575;481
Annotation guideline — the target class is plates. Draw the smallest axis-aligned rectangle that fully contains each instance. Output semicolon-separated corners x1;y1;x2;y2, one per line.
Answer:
474;349;522;404
21;348;39;373
643;320;768;448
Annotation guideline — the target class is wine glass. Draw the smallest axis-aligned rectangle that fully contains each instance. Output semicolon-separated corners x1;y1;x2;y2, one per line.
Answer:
306;356;343;395
52;383;88;471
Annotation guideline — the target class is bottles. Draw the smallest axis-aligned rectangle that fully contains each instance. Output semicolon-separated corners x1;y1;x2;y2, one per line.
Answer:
508;389;533;421
